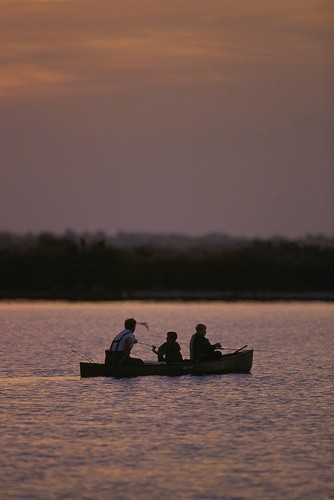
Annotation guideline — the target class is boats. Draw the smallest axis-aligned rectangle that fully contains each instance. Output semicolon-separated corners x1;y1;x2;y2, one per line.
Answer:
80;350;254;378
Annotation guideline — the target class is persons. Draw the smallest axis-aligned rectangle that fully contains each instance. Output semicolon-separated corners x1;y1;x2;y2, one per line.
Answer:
151;332;183;361
189;331;194;360
104;318;145;366
193;324;222;359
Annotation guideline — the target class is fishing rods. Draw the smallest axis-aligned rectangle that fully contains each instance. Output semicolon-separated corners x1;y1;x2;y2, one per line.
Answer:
215;348;271;351
136;341;159;349
56;334;94;363
